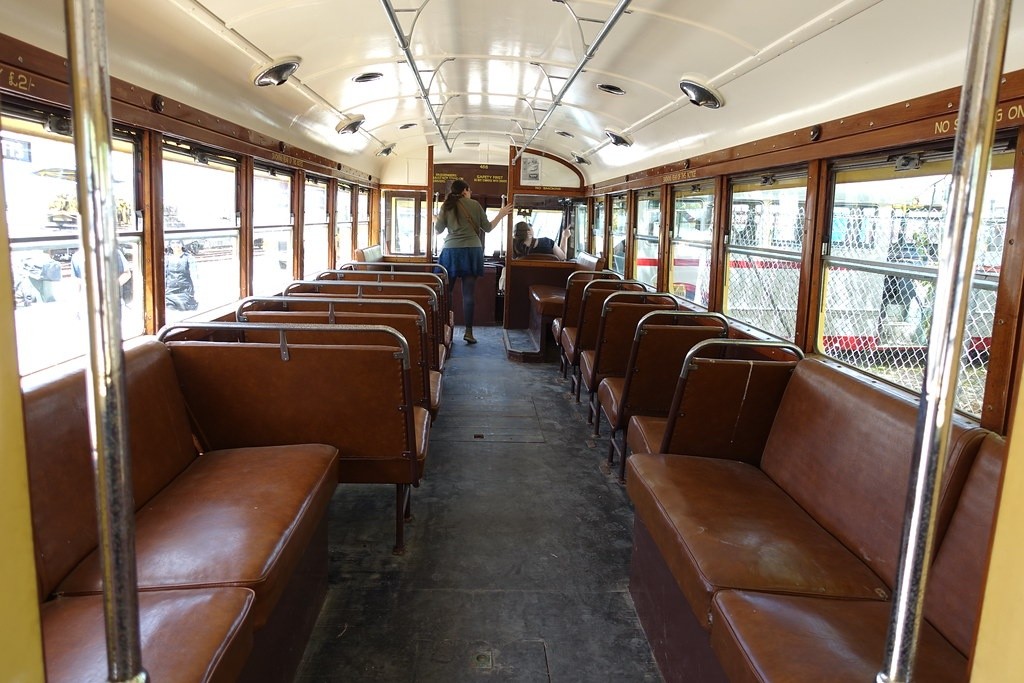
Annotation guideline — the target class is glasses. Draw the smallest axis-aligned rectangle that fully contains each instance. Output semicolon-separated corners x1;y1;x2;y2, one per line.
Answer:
526;221;533;226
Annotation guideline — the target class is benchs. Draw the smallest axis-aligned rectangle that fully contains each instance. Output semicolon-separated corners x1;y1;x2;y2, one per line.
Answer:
618;352;1010;683
526;251;606;349
156;253;456;560
15;333;340;681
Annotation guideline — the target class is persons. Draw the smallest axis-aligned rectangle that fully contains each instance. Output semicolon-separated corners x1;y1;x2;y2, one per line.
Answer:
433;179;512;343
163;239;198;312
69;242;132;320
513;222;572;261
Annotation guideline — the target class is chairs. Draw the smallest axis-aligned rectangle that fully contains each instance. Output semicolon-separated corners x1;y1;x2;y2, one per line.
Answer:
562;279;651;400
577;288;679;434
550;269;623;381
624;332;807;483
592;310;733;478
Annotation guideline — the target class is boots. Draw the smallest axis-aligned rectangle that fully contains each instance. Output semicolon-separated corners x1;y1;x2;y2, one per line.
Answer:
462;327;477;342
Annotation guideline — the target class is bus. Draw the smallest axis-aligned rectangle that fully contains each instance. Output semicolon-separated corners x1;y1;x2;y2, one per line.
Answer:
635;196;1008;363
